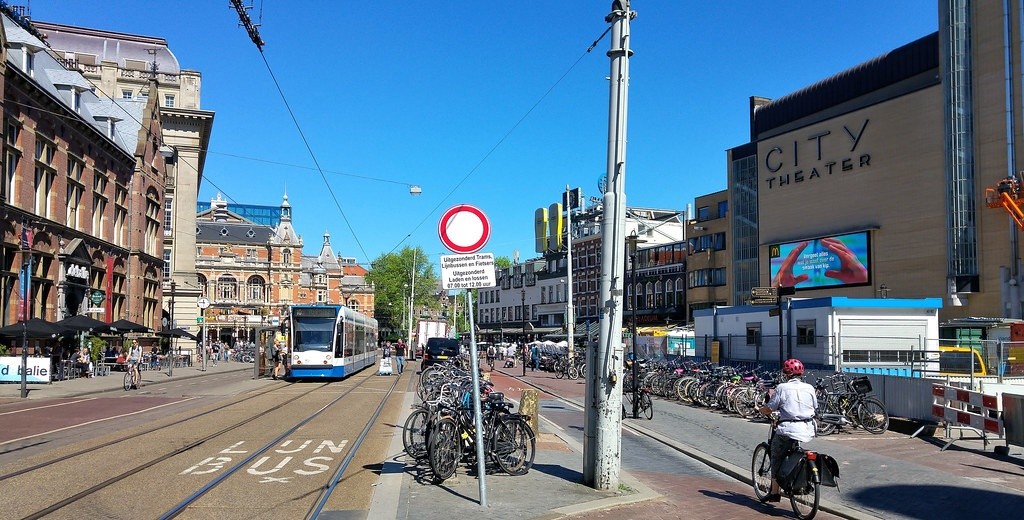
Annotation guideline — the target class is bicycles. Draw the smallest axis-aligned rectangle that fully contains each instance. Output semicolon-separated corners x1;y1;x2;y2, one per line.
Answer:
623;354;891;434
123;359;141;391
486;353;496;371
401;342;586;484
750;407;822;519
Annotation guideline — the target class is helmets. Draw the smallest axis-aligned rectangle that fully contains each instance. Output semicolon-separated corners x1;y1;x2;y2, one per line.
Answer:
783;359;804;375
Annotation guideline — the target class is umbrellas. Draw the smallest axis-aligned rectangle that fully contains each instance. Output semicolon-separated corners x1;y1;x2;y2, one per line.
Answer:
0;314;198;354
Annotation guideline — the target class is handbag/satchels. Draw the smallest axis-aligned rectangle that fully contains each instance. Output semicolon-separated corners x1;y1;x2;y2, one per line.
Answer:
776;450;807;490
807;452;841;487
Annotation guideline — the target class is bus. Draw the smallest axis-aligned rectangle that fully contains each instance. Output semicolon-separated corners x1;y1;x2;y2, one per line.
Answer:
281;303;379;378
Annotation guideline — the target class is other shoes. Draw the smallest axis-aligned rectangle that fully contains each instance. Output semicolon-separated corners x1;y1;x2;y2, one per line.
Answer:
766;493;780;503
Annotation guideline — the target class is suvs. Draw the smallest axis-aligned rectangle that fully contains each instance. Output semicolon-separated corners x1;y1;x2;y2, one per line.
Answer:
420;337;460;371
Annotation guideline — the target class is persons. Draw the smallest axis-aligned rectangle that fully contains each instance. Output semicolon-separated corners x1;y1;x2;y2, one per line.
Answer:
771;238;868;287
41;338;541;386
755;360;817;503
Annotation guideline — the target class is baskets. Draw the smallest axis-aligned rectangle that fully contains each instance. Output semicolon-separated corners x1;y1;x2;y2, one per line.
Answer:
853;376;872;394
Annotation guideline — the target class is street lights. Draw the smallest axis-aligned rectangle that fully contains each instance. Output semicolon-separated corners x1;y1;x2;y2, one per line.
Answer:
626;230;649;419
170;279;176;348
520;289;527;376
